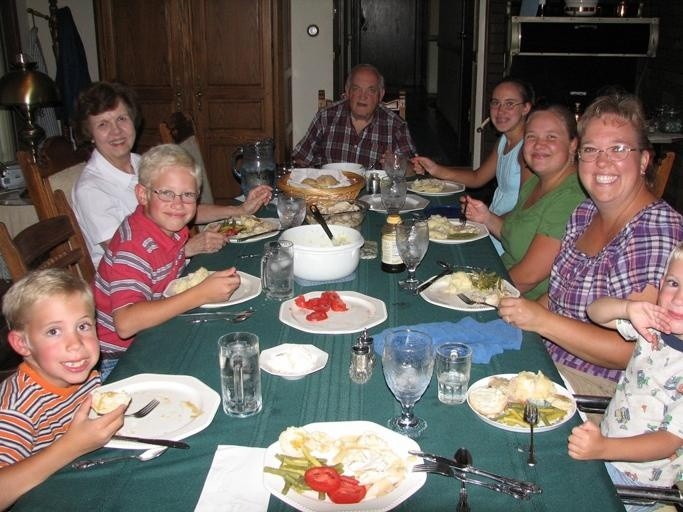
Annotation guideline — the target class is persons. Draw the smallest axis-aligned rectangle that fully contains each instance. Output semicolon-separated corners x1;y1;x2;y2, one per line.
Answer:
564;238;683;511
494;88;683;429
68;79;277;273
280;60;426;180
457;99;588;303
89;139;243;388
0;267;129;512
409;72;540;259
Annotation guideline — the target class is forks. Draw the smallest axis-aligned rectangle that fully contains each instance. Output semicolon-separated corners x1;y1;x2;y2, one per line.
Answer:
416;160;432;179
96;398;159;418
412;461;529;501
456;292;497;310
455;201;468;231
522;404;538;467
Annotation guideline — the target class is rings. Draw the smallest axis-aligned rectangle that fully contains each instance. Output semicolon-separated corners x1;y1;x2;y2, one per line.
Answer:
473;201;484;209
506;316;511;323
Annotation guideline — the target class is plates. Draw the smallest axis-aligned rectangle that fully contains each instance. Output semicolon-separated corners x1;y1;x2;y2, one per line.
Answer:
321;163;360;174
203;218;281;243
263;421;427;512
465;373;577;432
406;179;465;196
416;271;520;312
429;218;489;244
87;373;220;450
259;343;329;380
278;290;387;334
161;270;261;309
425;204;461;219
358;193;430;215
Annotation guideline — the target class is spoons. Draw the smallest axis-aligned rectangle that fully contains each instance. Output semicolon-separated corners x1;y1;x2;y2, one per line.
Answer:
453;447;473;512
191;315;248;324
70;445;168;470
436;260;488;271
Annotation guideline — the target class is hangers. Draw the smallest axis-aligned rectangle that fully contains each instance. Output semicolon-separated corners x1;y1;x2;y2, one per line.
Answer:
29;7;38;36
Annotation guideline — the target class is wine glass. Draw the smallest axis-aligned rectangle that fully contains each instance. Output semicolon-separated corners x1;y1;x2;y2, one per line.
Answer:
395;219;429;288
381;329;435;439
380;175;407;215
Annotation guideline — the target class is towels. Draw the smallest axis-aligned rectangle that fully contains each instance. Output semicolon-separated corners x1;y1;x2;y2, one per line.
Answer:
368;316;525;366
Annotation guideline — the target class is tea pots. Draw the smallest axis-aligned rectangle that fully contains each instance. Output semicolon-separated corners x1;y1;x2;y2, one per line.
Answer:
229;141;277;197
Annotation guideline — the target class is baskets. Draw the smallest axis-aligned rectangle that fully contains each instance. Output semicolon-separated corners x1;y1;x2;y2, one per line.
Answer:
277;170;365;214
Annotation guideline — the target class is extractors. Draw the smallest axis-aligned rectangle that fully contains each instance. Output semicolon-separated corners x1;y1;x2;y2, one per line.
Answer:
508;17;660;59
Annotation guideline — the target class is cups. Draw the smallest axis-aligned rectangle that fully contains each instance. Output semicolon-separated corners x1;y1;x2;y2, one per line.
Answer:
276;192;306;229
434;342;472;405
217;331;262;419
383;152;407;180
260;239;294;302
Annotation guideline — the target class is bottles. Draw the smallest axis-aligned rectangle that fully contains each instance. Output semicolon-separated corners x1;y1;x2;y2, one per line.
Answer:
644;102;683;133
368;172;379;195
348;337;371;384
356;328;377;369
379;214;406;273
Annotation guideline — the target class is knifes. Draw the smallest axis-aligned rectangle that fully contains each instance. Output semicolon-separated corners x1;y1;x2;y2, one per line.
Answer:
417;270;452;292
407;450;543;494
111;435;190;449
176;310;256;316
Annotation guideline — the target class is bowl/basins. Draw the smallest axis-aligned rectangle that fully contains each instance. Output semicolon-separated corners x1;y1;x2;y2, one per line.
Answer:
278;225;365;281
304;199;369;229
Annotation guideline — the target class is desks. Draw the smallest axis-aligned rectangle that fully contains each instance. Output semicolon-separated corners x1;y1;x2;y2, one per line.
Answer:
0;190;50;284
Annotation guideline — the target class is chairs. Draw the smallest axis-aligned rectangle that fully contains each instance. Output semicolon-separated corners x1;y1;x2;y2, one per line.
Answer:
643;150;676;201
571;394;683;512
0;187;98;295
158;109;217;237
16;134;99;283
317;87;407;121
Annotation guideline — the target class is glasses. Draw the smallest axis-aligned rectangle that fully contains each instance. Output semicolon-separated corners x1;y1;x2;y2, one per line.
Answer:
489;100;523;109
154;189;199;204
577;145;643;162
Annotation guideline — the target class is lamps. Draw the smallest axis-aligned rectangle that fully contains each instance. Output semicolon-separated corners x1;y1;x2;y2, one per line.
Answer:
1;50;63;199
